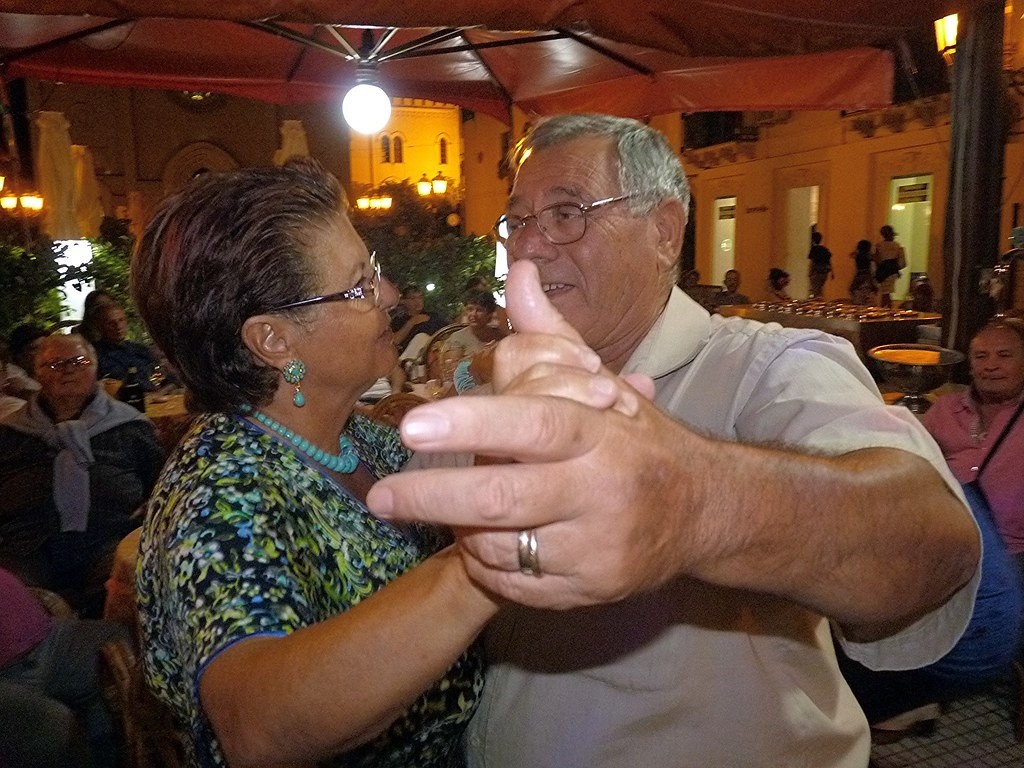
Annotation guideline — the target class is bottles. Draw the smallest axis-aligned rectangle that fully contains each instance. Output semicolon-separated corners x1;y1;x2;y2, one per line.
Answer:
121;366;144;414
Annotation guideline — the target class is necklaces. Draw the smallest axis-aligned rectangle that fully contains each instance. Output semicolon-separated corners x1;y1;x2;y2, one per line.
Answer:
246;410;359;474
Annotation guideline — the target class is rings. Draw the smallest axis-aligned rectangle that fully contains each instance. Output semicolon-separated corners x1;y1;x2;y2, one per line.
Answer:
519;526;540;579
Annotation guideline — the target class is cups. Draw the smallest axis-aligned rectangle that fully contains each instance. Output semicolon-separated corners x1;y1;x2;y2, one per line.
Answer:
442;358;454;383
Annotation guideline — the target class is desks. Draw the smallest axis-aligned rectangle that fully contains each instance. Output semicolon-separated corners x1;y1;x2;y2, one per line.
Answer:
102;526;145;621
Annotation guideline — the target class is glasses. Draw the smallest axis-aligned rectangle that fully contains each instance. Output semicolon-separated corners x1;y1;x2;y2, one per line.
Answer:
492;189;649;246
38;354;94;371
265;249;382;315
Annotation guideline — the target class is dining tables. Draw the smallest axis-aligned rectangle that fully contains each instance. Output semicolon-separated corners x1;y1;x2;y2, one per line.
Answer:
140;391;188;446
713;299;945;381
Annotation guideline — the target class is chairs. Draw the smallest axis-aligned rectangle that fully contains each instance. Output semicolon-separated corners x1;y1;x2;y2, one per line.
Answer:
403;318;500;384
370;391;431;428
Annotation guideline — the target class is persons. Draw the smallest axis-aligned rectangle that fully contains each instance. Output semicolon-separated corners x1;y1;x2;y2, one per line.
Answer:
365;114;982;768
439;290;513;397
456;278;506;325
130;162;655;768
0;291;191;768
686;225;936;321
873;323;1024;731
387;281;450;361
357;363;408;406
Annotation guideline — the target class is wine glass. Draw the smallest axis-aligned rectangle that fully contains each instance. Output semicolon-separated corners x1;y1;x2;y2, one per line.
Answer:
147;362;167;405
